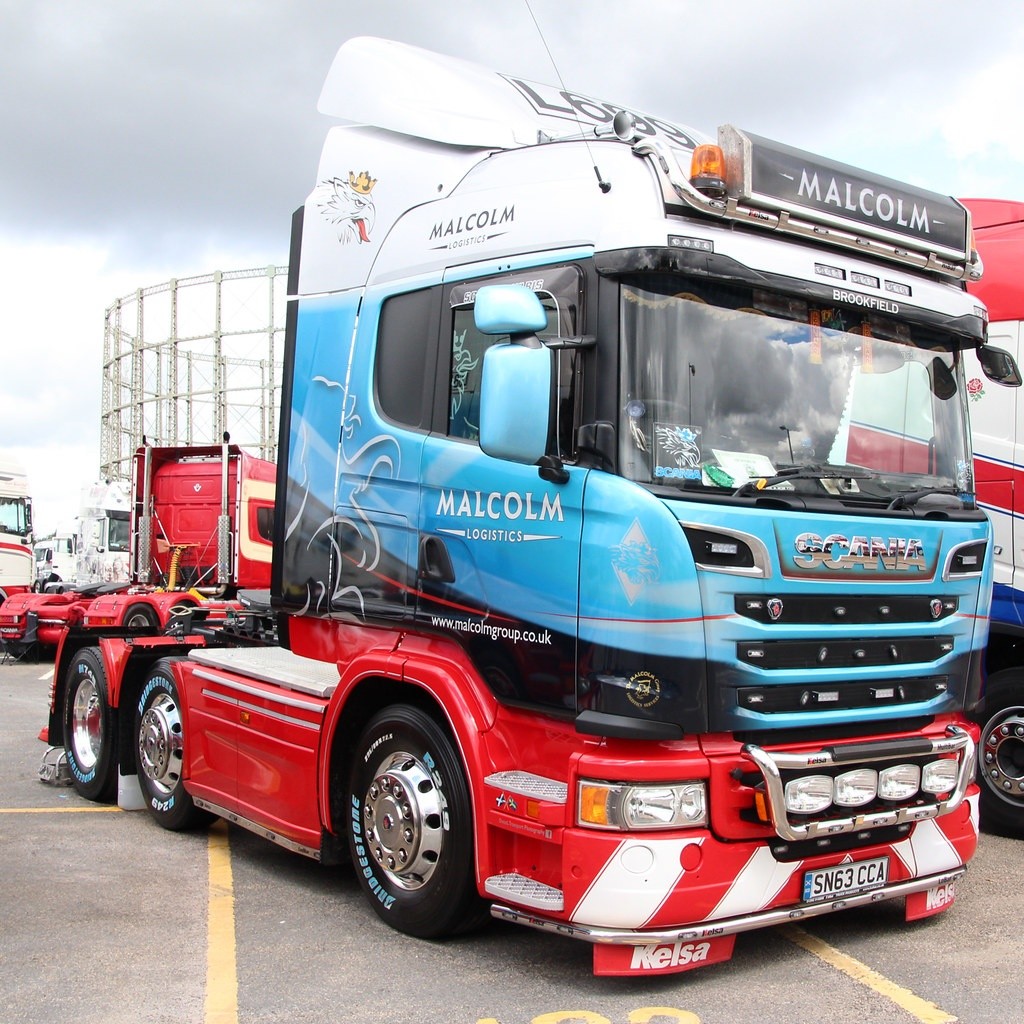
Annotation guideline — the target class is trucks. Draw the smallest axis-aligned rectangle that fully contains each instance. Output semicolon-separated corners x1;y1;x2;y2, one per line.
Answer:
33;518;82;593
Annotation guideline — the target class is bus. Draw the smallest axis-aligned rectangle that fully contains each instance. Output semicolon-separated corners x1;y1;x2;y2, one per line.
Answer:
0;470;37;607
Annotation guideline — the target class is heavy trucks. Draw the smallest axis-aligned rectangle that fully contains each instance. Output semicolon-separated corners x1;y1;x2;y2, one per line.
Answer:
34;32;1024;978
